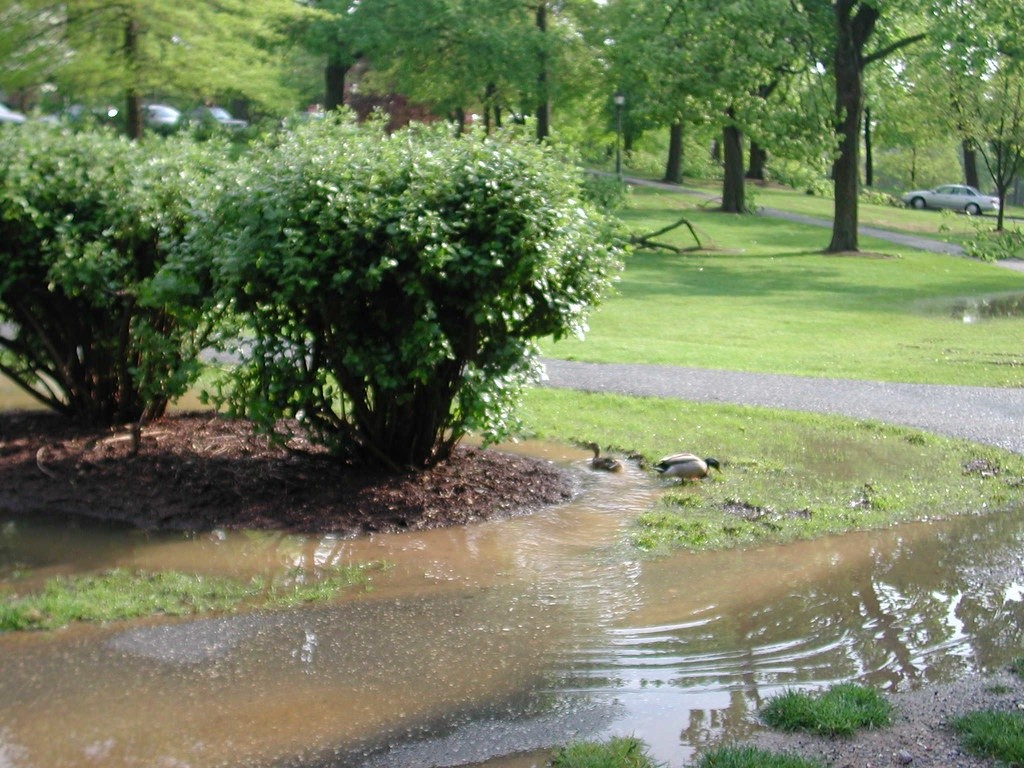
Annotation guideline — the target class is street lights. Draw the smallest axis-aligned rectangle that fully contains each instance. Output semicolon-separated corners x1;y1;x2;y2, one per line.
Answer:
614;88;624;172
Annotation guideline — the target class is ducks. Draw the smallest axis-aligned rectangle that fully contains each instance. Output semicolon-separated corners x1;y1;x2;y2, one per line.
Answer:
649;452;721;487
584;442;622;472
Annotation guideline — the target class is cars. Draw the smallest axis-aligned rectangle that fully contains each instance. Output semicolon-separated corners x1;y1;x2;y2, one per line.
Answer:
901;185;1000;215
141;105;180;127
191;106;248;129
